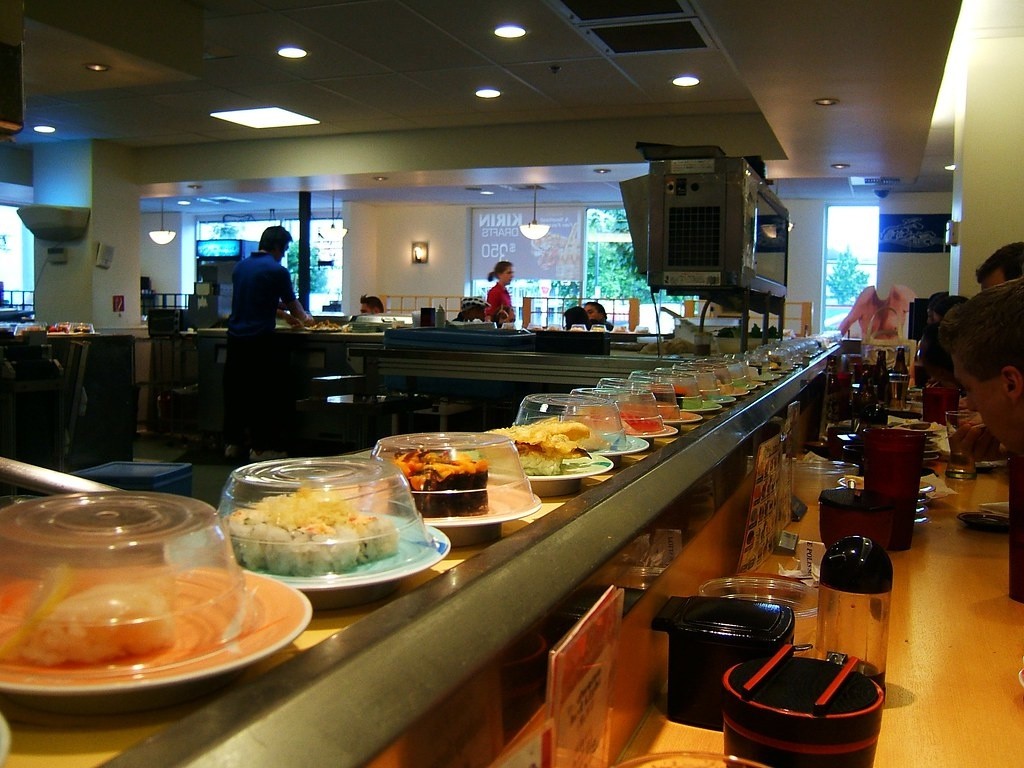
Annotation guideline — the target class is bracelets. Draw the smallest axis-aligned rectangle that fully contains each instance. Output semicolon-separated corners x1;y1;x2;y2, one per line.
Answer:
303;314;308;322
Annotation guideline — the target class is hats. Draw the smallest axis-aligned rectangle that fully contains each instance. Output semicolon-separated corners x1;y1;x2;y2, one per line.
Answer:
460;298;491;312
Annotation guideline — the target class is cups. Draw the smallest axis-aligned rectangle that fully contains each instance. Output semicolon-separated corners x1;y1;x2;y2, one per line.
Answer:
792;457;860;564
922;387;960;427
945;411;983;480
862;427;926;552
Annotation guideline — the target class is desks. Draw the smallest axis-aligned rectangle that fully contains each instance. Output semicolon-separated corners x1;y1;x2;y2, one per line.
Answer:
519;185;549;239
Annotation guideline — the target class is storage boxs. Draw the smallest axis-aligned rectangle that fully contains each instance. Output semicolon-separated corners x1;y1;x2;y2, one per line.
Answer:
69;460;192;499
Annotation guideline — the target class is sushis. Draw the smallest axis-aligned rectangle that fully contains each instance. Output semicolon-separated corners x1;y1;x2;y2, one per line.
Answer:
22;581;175;668
227;515;398;577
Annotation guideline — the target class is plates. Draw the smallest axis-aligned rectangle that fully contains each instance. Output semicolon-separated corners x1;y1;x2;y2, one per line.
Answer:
16;330;101;336
0;563;314;714
415;484;542;548
957;512;1009;533
621;340;839;443
590;436;650;469
839;421;942;526
162;510;452;610
486;453;614;497
306;328;342;333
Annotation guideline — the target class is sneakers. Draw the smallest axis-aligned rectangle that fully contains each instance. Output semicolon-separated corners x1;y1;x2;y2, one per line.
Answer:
225;444;239;459
248;448;288;462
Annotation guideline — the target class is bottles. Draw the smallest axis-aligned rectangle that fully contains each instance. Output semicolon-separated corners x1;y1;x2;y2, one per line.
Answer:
815;535;894;697
893;347;909;400
871;350;893;408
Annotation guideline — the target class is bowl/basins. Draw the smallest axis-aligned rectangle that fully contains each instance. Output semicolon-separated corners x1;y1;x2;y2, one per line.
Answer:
696;576;819;659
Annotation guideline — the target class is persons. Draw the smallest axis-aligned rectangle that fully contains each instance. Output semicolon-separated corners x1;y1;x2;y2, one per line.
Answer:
485;261;514;321
583;302;613;331
937;278;1024;464
222;225;315;461
916;240;1024;449
564;307;590;330
491;306;516;329
360;294;385;314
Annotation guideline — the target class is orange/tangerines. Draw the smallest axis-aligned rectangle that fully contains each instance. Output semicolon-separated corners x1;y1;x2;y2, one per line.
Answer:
0;564;73;662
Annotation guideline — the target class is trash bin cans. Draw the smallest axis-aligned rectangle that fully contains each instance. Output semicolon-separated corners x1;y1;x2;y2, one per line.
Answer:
69;461;193;497
652;595;795;732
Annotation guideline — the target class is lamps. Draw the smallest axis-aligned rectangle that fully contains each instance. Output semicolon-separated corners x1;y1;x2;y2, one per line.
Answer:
149;199;176;244
